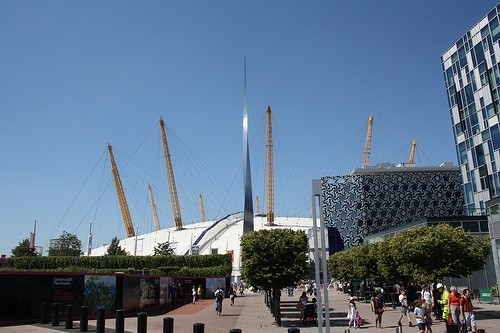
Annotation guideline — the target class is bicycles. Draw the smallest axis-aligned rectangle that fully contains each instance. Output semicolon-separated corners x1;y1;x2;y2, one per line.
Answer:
215;296;224;316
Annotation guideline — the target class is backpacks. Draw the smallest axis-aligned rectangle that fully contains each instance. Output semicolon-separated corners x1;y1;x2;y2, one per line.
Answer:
216;288;223;301
355;314;363;326
306;305;315;313
454;296;466;325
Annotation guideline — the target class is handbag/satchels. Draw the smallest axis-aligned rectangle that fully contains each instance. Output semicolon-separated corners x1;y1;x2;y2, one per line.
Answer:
296;301;304;308
446;316;455;325
231;294;237;300
378;308;385;313
426;317;433;323
458;319;467;332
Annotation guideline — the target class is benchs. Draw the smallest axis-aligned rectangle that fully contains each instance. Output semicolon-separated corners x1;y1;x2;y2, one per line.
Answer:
280;301;350;325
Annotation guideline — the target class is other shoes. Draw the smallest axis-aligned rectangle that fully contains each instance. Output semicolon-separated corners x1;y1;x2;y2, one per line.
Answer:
409;322;414;327
435;316;443;319
398;321;403;326
379;325;383;329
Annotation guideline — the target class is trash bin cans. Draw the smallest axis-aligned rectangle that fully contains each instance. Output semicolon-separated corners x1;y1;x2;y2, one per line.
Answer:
477;288;493;304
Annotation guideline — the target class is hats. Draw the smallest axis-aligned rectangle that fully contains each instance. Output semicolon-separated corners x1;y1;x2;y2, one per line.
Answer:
311;298;317;303
421;283;429;290
349;297;353;300
436;283;444;289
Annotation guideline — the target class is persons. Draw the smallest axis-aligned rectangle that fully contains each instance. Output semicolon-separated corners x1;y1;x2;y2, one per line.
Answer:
214;287;225;314
373;289;385;328
398;288;414;327
436;283;477;333
239;284;244;295
299;292;318;321
490;286;496;305
347;297;358;328
229;287;237;305
306;281;316;295
473;288;482;304
170;284;184;305
421;283;443;319
414;299;433;333
393;280;400;306
192;286;197;304
196;285;203;302
